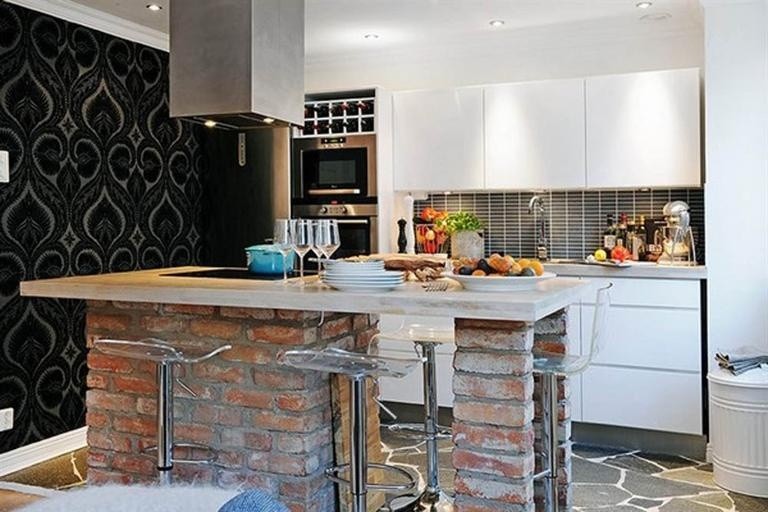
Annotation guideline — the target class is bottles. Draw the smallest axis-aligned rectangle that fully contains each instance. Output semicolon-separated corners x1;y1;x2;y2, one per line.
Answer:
292;99;373;134
601;212;650;264
397;219;407;253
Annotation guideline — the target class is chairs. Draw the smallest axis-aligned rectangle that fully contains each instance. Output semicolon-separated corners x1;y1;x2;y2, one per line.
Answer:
531;282;612;512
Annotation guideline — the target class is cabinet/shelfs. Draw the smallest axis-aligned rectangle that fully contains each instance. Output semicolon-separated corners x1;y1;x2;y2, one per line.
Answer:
586;67;701;191
391;84;484;192
484;78;585;193
565;281;581;422
581;276;702;438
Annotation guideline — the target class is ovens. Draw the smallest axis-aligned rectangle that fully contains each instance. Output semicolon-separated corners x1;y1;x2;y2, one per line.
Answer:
288;131;377;276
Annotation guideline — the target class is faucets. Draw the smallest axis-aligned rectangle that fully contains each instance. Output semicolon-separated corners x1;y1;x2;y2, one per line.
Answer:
528;196;545;240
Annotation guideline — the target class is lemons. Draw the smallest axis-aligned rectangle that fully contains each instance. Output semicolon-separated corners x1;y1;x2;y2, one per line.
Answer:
595;250;607;261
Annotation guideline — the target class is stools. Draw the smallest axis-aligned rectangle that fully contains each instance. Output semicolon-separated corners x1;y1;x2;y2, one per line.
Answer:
277;346;419;511
94;337;233;487
376;314;457;511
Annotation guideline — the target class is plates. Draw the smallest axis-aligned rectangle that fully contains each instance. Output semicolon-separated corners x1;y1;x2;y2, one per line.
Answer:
320;259;406;293
439;269;557;294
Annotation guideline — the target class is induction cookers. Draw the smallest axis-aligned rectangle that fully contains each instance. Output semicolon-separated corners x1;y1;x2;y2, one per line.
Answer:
160;267;320;281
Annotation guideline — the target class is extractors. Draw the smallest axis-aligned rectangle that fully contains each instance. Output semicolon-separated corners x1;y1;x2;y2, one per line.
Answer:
166;1;308;130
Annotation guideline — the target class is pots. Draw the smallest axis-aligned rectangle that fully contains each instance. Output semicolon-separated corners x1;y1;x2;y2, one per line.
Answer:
244;238;295;274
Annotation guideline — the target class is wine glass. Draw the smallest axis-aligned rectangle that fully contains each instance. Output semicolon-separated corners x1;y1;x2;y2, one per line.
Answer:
274;218;342;286
